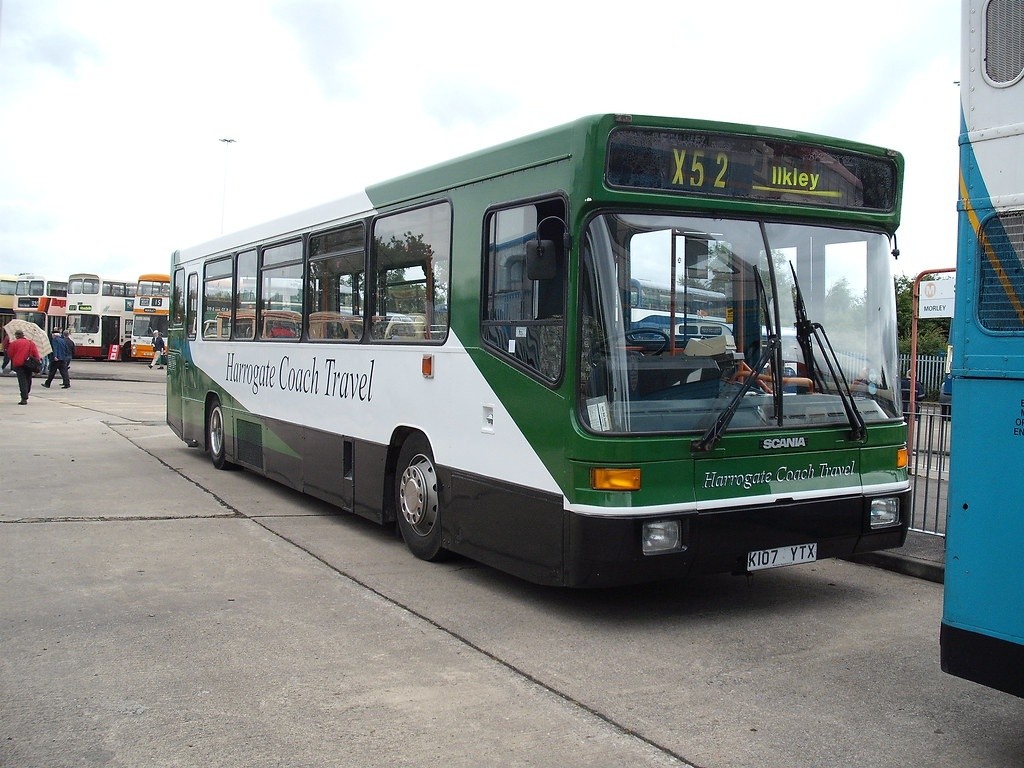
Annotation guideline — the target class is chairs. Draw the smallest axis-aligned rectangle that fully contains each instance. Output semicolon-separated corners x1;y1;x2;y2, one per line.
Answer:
538;317;602;388
203;308;445;341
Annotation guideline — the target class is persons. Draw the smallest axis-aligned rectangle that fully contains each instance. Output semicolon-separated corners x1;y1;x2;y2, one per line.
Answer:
0;327;76;389
147;330;164;369
7;331;40;405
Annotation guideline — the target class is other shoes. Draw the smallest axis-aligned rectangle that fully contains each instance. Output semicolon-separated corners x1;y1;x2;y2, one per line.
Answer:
0;368;4;373
157;367;163;369
18;402;27;405
11;370;17;374
60;383;64;386
42;384;50;388
61;386;69;388
149;365;152;368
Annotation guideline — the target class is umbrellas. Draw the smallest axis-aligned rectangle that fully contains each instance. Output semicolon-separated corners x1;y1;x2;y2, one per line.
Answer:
3;319;53;359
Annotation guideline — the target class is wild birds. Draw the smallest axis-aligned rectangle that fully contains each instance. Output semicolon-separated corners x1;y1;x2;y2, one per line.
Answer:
218;137;236;144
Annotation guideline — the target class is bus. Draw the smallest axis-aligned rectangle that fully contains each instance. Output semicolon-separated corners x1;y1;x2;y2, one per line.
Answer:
940;0;1024;700
628;278;727;340
130;274;363;365
166;114;911;589
65;273;170;361
13;279;120;343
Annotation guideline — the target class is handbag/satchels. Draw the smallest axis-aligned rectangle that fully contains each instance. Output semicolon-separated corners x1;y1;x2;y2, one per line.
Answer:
23;356;43;373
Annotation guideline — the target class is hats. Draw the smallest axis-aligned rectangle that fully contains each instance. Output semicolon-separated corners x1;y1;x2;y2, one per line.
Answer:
52;331;60;333
153;330;159;334
